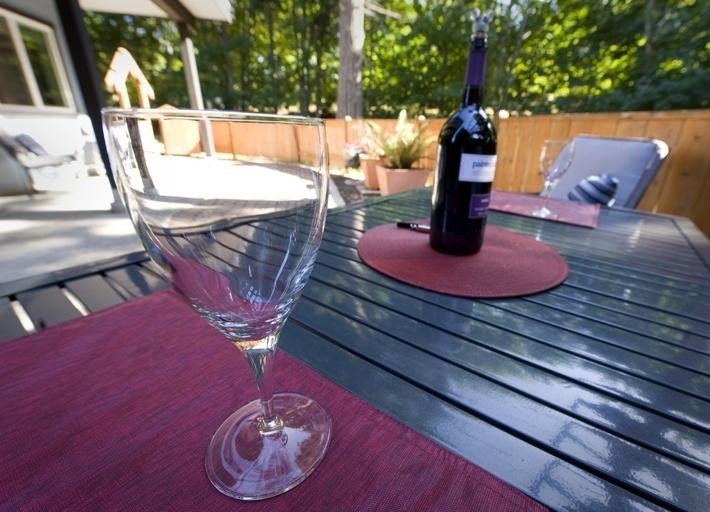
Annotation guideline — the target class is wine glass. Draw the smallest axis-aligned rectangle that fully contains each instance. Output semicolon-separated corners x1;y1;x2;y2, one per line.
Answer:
535;139;578;201
100;106;333;501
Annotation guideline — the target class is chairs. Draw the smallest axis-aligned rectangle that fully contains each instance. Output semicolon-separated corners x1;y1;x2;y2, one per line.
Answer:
538;131;671;211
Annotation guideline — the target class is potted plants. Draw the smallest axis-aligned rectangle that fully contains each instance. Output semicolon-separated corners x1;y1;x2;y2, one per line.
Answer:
346;110;439;197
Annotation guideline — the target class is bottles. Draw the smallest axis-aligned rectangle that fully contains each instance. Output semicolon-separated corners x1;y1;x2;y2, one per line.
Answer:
426;12;504;256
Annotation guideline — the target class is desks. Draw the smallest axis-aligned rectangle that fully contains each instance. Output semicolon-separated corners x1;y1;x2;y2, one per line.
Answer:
1;184;710;510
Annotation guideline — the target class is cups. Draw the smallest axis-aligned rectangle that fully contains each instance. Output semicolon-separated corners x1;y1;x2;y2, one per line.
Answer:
564;174;622;210
